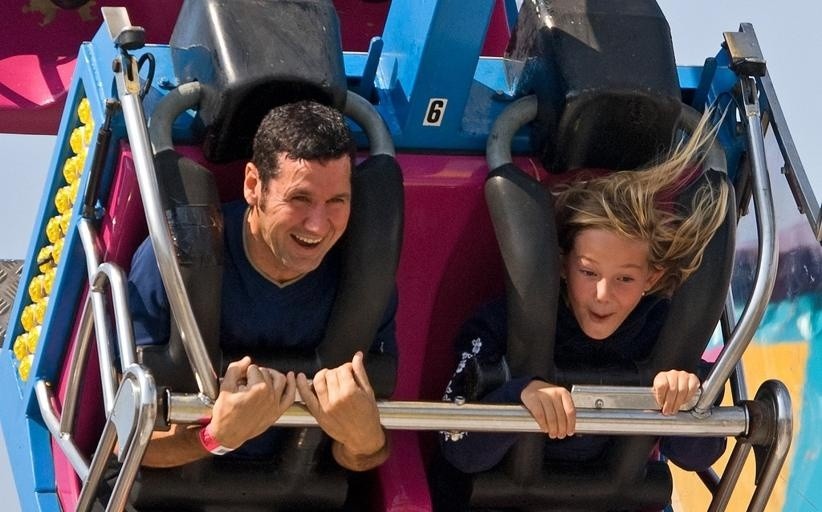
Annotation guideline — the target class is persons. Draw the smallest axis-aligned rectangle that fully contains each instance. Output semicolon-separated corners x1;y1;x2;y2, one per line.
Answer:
89;101;398;512
429;100;727;511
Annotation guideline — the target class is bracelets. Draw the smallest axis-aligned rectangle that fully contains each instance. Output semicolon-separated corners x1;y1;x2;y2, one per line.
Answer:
198;428;234;456
